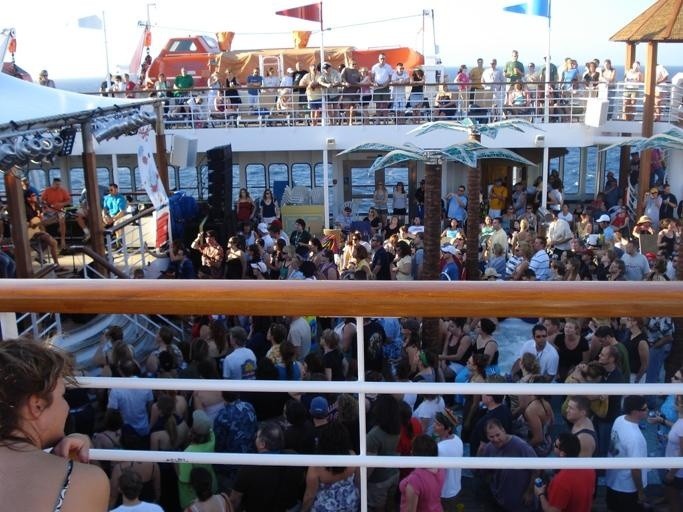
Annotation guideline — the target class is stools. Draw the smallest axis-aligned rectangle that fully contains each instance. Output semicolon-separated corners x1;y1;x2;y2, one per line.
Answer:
30;236;50;268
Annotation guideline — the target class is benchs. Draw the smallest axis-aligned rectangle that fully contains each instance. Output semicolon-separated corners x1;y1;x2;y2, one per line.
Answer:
41;218;92;240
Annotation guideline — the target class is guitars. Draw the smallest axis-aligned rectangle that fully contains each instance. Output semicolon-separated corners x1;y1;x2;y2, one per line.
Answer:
27;216;57;241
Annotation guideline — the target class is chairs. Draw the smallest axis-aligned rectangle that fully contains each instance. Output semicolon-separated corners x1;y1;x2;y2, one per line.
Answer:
123;206;170;255
341;201;359;222
444;218;463;229
281;184;323;210
187;90;300;126
252;197;260;229
474;89;494;109
65;389;108;439
639;234;661;255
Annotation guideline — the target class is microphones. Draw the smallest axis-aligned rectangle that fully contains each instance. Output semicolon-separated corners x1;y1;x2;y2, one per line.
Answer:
37;196;42;208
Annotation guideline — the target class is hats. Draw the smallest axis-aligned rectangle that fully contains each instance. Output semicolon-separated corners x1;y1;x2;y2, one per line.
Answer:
595;214;611;223
192;408;211;434
309;396;328;414
636;215;653;225
441;245;457;256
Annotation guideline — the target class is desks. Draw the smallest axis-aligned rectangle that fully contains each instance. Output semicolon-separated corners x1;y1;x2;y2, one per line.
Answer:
280;203;325;240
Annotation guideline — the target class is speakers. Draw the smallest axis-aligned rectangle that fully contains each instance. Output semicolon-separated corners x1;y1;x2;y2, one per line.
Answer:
207;145;232;225
583;98;610;128
170;135;198;169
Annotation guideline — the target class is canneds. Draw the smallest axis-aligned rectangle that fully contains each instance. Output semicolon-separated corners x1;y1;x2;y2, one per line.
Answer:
649;411;656;418
535;477;543;487
